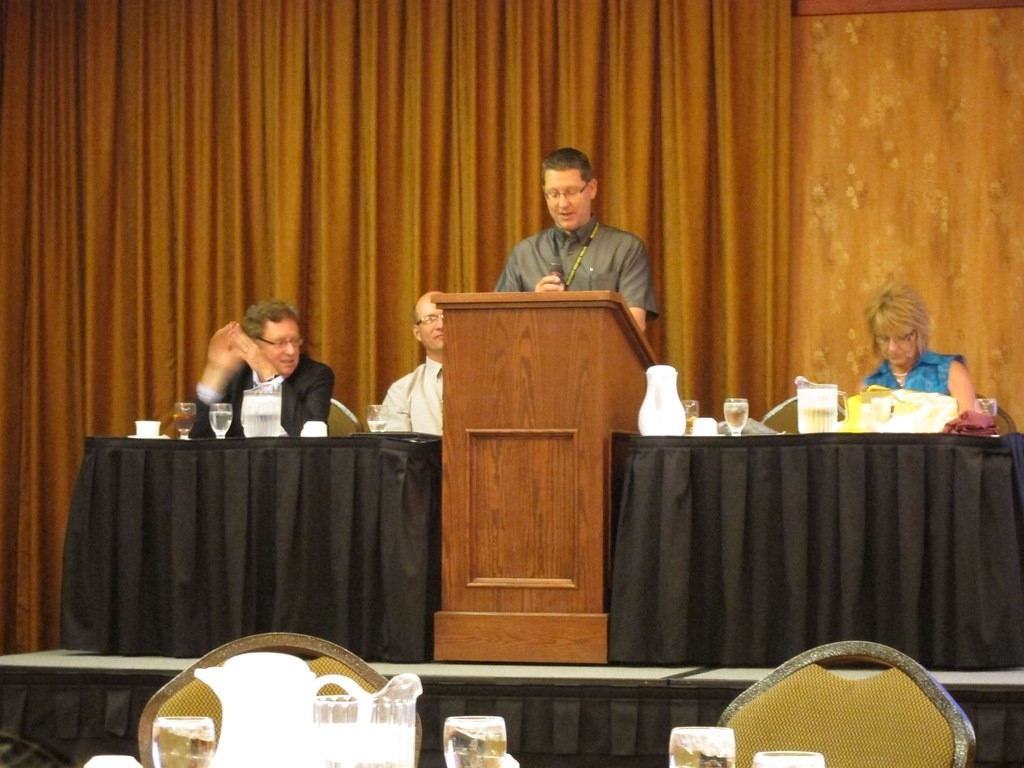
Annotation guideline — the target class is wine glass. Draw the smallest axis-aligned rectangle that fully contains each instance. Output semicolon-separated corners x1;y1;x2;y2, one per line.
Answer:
723;398;749;436
174;402;196;440
209;404;232;439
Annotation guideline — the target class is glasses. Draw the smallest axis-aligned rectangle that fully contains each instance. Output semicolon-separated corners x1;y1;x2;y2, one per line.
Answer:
541;180;592;200
416;315;442;325
876;330;915;345
250;333;304;347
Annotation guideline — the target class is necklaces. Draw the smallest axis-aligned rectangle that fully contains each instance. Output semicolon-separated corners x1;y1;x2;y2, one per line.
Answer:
893;373;906;377
897;379;903;387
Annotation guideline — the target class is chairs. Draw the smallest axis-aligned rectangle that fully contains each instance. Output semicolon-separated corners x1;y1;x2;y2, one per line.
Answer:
324;397;363;436
139;632;423;768
758;394;846;429
972;392;1017;436
716;639;976;768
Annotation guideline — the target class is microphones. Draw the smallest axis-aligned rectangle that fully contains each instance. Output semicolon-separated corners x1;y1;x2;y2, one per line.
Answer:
548;258;562;279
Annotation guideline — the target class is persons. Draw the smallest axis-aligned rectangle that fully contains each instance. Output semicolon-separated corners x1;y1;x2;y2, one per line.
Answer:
494;148;660;333
378;291;443;436
856;283;976;414
178;299;334;437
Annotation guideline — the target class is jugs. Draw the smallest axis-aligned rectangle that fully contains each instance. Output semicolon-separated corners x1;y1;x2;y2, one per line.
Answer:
794;376;849;434
241;390;281;437
192;652;315;768
314;673;422;768
638;365;686;436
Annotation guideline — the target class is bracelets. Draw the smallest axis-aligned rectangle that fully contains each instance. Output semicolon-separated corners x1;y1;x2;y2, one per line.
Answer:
266;374;280;382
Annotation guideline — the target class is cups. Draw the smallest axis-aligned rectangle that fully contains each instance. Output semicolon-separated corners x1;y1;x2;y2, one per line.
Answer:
872;398;892;425
680;400;699;436
976;398;999;437
692;418;718;436
135;420;161;438
301;421;329;437
366;405;387;432
669;726;824;767
444;716;506;768
153;717;214;767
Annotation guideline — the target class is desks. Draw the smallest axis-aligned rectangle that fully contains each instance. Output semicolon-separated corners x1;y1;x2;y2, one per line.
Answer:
607;434;1024;670
61;433;441;665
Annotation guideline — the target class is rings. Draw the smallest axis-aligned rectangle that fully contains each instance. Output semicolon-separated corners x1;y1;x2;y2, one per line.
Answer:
244;348;248;352
